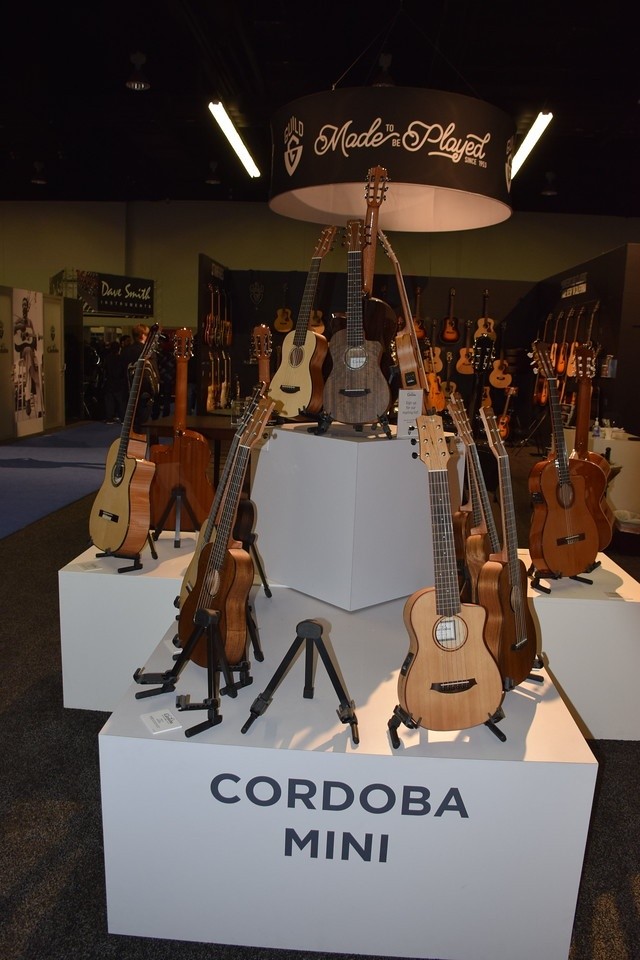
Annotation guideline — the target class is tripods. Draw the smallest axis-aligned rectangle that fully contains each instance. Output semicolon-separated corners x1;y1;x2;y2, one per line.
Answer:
95;434;601;750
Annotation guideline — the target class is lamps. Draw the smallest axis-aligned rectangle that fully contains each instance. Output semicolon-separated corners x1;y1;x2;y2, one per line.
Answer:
126;53;151;90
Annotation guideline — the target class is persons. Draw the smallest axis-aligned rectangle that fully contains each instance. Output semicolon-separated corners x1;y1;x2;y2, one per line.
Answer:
14;297;42;418
90;324;175;424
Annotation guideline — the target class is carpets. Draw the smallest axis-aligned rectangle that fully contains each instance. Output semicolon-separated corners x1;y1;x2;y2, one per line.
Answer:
0;421;174;541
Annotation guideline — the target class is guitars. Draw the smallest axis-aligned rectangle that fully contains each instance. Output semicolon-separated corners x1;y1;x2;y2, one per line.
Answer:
87;163;612;732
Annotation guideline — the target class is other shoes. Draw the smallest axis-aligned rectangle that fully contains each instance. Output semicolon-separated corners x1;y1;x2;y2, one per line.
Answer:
24;399;31;416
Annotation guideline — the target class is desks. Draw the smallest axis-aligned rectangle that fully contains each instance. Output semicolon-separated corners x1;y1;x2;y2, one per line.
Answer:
142;415;241;446
